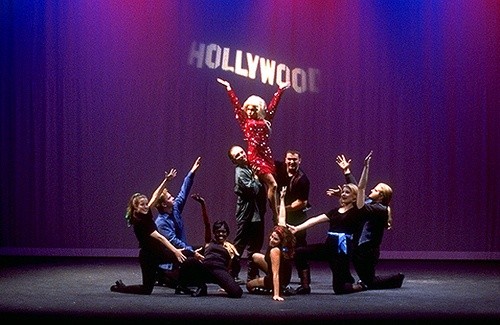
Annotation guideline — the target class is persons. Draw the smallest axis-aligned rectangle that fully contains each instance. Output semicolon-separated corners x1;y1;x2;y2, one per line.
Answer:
228;144;267;281
326;154;405;290
215;77;289;220
275;150;312;296
110;169;194;295
247;186;296;301
287;151;373;294
153;156;201;288
180;194;247;299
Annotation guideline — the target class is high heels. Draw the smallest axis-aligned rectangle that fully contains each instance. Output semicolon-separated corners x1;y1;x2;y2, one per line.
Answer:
174;283;194;295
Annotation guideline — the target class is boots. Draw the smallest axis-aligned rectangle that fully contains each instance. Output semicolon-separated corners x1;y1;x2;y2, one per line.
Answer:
344;280;369;293
295;268;312;295
376;273;405;288
253;287;296;294
111;279;142;295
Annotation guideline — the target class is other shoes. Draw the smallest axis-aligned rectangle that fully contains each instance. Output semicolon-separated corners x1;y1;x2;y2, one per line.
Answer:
230;272;245;285
190;285;207;297
